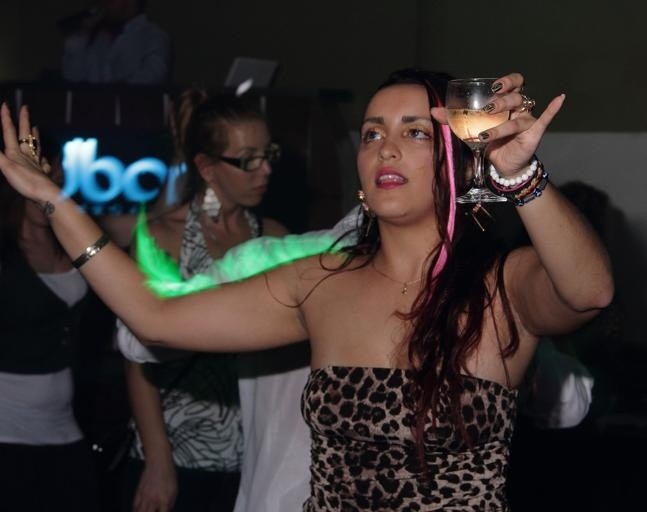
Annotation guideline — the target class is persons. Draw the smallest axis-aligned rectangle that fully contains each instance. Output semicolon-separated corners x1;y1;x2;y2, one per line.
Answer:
1;67;617;512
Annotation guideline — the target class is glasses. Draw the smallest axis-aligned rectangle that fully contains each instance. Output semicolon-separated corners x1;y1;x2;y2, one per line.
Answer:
219;144;284;172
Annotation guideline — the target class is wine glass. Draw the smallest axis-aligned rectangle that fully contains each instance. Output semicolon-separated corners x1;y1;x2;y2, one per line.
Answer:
442;75;513;204
